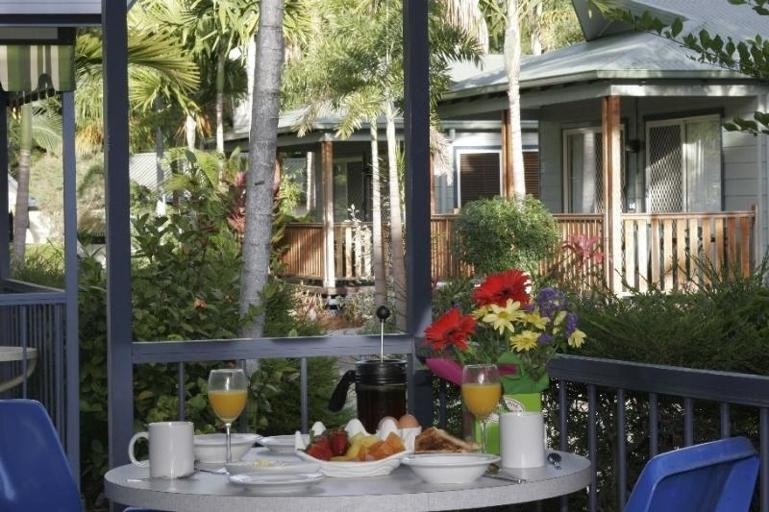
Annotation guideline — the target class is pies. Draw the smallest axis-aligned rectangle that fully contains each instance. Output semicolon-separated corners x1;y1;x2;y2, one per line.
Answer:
414;427;483;451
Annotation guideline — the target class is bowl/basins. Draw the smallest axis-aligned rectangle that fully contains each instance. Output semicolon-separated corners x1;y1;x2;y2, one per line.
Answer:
400;452;502;485
194;433;264;463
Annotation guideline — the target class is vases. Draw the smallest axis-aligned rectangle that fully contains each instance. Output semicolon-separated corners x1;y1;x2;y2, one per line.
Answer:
472;392;542;456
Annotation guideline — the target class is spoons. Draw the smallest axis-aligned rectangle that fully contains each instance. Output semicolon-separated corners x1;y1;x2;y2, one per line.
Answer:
489;463;527;483
126;472;196;484
547;452;562;471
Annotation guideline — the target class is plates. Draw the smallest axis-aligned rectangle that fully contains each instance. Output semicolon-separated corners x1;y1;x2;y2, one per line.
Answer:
295;420;421;477
257;434;312;454
229;471;325;495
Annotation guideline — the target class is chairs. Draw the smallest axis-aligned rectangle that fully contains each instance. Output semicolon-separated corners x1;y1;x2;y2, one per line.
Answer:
621;435;760;512
0;399;87;512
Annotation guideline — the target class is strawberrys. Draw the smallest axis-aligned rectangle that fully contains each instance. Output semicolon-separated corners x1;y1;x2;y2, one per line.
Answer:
306;428;345;460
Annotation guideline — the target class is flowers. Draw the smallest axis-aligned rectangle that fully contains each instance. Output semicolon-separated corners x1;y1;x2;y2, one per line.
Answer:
418;270;588;394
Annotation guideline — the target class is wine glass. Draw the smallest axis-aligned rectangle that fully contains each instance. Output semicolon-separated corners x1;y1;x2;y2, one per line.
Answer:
207;369;249;474
461;365;501;452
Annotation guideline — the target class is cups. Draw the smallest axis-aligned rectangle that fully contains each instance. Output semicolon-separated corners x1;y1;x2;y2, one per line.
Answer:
128;421;195;478
499;413;546;470
